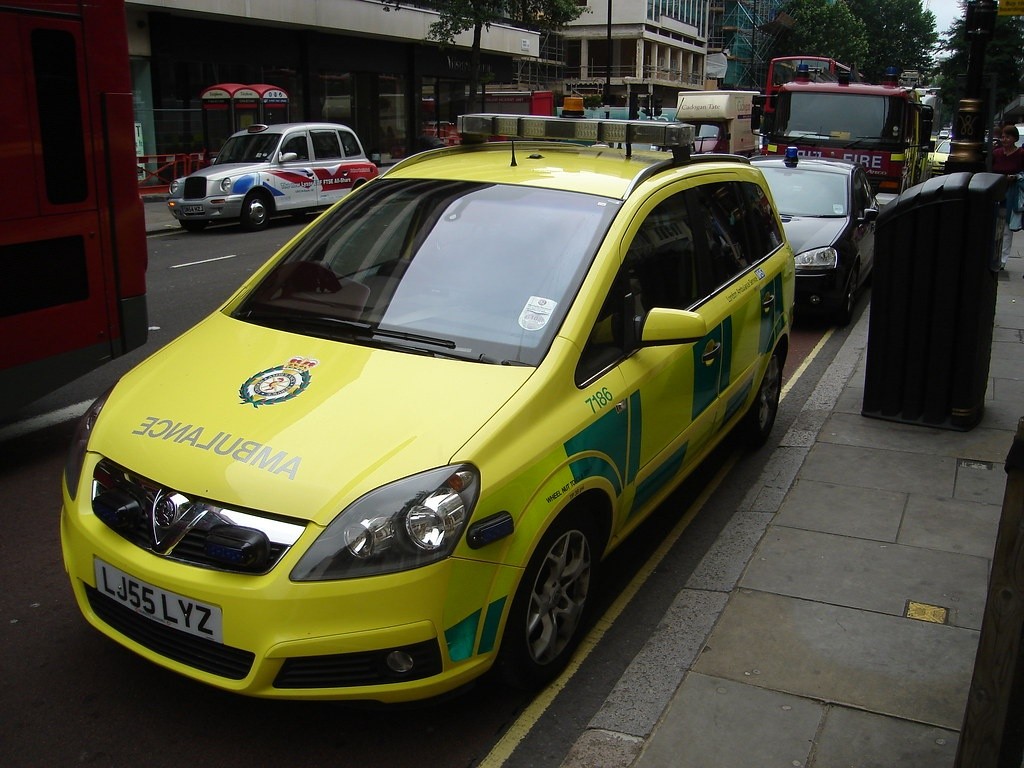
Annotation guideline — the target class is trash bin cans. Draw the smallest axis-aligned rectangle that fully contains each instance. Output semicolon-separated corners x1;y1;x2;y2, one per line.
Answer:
859;169;1009;432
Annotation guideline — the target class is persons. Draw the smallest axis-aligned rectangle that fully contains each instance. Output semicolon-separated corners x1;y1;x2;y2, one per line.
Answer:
624;264;667;350
709;226;742;273
744;196;775;259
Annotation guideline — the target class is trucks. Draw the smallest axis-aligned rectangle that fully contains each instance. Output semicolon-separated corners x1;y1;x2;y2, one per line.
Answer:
667;89;760;158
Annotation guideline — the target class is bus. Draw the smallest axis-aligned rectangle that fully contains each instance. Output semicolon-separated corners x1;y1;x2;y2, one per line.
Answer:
765;57;866;117
0;0;154;419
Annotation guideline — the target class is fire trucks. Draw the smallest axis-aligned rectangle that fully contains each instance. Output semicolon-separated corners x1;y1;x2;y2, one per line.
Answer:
750;55;936;197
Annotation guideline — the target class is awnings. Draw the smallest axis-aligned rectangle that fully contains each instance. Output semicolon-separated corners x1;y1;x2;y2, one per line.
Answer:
465;94;530;102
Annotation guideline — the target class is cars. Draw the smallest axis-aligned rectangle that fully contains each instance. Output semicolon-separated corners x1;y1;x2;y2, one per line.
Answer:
747;146;881;326
462;91;677;151
932;139;951;175
938;130;949;139
163;120;379;233
57;106;796;705
928;137;935;165
